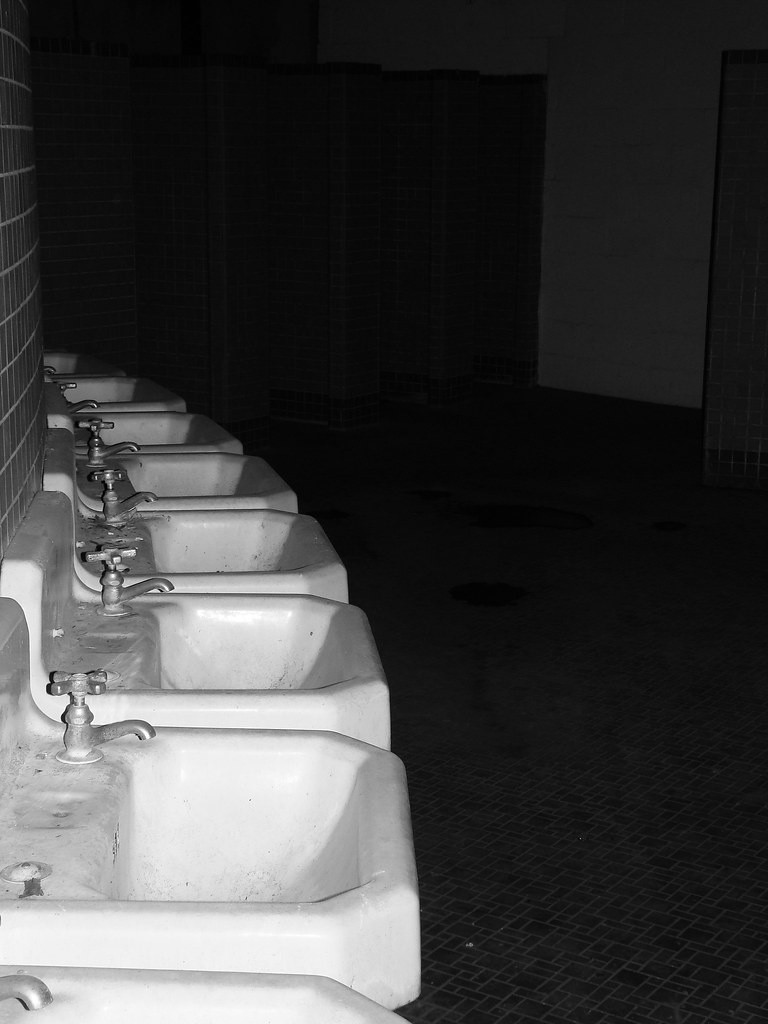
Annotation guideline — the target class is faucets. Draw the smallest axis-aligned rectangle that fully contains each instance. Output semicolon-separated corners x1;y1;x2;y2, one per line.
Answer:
50;667;156;757
0;974;54;1012
79;416;142;464
54;382;100;413
84;538;174;611
43;366;56;374
90;469;159;525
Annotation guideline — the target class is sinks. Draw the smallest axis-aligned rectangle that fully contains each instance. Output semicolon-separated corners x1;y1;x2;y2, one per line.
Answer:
54;376;187;413
90;410;244;454
140;508;350;603
121;452;300;511
151;594;393;750
76;720;423;1011
1;963;414;1024
